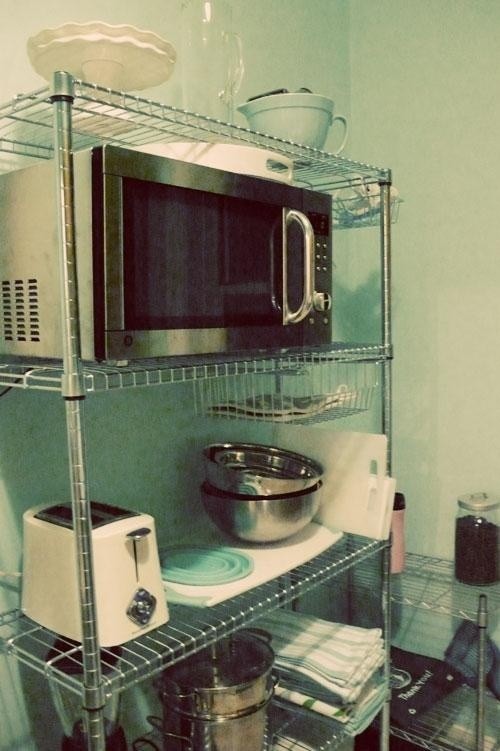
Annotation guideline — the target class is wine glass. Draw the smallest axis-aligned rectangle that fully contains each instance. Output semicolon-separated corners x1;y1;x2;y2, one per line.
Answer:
217;33;245;143
180;0;212;134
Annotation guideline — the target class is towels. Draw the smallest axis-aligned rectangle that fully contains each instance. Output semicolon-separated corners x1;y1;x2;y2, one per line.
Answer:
243;608;391;738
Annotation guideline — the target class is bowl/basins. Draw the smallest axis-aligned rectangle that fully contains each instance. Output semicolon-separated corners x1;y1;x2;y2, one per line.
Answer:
202;442;325;495
200;481;323;544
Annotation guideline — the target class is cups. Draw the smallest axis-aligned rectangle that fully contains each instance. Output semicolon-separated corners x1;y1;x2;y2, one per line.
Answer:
236;95;349;167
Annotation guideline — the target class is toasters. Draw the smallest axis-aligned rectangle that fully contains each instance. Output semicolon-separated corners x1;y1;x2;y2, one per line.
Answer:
21;498;166;648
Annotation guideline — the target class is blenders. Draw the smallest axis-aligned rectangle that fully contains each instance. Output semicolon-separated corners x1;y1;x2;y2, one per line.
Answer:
46;634;128;751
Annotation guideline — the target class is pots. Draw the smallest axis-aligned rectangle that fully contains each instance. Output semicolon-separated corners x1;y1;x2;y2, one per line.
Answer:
161;670;281;751
158;628;281;715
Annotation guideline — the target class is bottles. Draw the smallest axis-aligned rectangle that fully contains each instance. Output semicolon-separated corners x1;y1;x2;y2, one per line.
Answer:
389;493;405;575
455;494;500;585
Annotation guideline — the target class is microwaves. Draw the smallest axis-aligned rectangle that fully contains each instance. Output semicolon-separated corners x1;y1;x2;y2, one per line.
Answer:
0;146;334;365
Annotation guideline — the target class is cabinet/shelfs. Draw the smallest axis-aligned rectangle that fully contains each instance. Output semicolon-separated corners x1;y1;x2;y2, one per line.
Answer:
1;73;403;751
278;533;500;750
